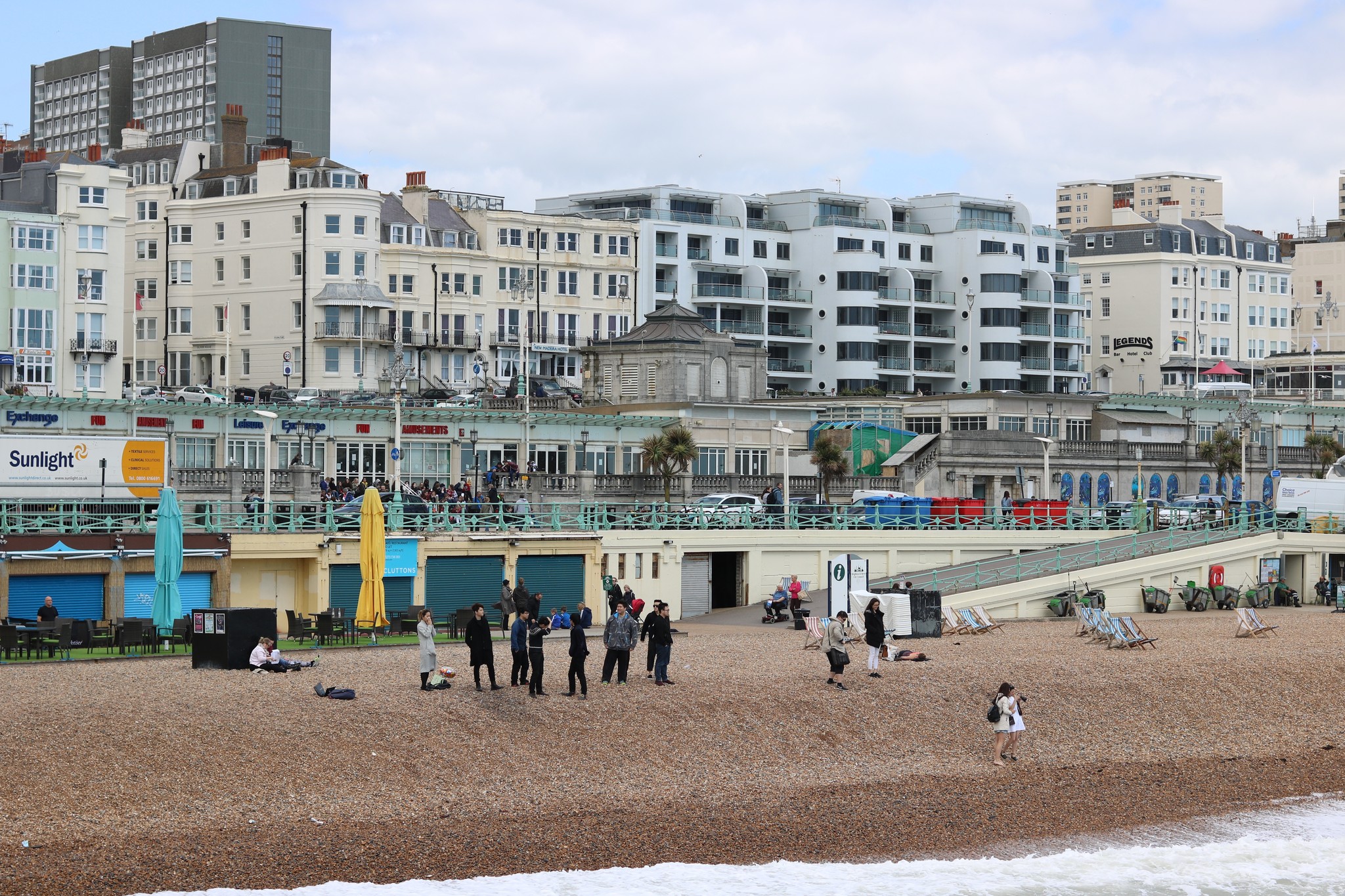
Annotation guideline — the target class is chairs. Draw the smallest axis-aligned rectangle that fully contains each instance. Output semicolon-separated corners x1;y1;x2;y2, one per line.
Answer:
789;580;813;603
957;609;995;636
327;607;346;640
462;606;475;637
882;622;896;644
1233;607;1268;640
971;605;1007;635
821;617;857;650
285;610;312;642
455;609;473;640
802;615;827;651
0;614;192;661
846;611;867;645
428;607;451;639
1244;606;1279;638
316;613;345;646
774;576;792;595
356;611;379;645
399;605;425;636
1071;601;1158;651
941;606;973;637
297;612;320;645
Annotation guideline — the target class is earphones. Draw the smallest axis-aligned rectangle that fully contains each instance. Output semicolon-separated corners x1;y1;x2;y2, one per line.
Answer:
625;608;626;610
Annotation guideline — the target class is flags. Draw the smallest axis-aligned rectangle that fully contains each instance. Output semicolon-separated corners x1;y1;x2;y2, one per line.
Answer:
1309;336;1321;355
1175;337;1187;344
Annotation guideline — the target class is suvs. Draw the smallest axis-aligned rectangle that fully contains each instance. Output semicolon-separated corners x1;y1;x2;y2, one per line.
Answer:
763;496;832;530
678;492;763;527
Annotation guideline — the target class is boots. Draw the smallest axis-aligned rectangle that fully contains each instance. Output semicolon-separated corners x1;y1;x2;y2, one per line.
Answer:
1294;599;1331;607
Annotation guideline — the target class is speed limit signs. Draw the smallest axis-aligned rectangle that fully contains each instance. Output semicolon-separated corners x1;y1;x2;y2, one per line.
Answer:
158;365;166;375
283;351;291;360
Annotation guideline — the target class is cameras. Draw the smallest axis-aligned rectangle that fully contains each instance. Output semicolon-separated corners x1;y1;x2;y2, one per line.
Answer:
610;596;614;602
1016;693;1027;702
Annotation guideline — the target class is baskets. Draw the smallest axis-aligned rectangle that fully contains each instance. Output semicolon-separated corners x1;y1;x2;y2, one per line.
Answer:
764;602;772;609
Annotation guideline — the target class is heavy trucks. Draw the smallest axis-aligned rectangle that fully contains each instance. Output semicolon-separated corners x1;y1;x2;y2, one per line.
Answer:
0;433;170;534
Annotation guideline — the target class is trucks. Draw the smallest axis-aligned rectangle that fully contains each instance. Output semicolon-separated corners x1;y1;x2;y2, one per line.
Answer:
1275;477;1345;532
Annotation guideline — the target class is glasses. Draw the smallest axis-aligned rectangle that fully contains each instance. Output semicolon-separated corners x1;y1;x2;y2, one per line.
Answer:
1319;578;1323;580
537;596;542;599
508;583;510;584
624;588;628;591
523;612;530;614
873;604;879;607
264;642;270;647
612;580;616;582
653;604;669;612
839;616;846;621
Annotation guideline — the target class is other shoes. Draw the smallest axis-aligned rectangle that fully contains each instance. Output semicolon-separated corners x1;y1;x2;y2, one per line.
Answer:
420;685;434;691
289;668;293;673
993;750;1018;767
474;673;675;700
826;672;882;691
268;670;274;673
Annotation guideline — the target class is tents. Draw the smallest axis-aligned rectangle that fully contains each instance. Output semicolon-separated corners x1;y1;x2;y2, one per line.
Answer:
1199;359;1245;383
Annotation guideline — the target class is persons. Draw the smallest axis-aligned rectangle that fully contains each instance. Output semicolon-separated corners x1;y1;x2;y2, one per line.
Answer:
270;380;275;386
527;615;552;700
1030;496;1035;499
601;600;639;685
892;583;900;591
787;574;802;622
991;682;1006;768
924;388;931;396
1207;498;1216;531
464;603;506;692
1000;491;1012;520
415;609;437;692
560;613;591;701
894;650;931;662
652;603;676;688
510;606;530;688
1314;577;1332;606
242;453;543;530
249;636;294;674
36;596;58;623
803;389;810;397
500;574;634;630
208;370;212;387
764;583;788;618
863;598;886;679
771;482;785;529
391;320;396;341
820;610;851;692
49;390;53;397
999;685;1026;762
266;635;321;668
830;387;837;397
640;600;664;681
1276;576;1302;608
759;486;776;530
917;388;923;397
904;581;913;590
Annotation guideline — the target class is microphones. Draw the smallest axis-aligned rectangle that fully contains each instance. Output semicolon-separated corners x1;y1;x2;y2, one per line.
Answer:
618;613;619;615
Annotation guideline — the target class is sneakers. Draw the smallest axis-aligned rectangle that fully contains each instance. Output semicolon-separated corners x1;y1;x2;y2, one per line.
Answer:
769;614;796;622
311;653;320;668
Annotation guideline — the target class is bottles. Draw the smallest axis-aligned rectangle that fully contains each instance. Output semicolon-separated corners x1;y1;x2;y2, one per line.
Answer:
332;608;334;618
338;608;341;618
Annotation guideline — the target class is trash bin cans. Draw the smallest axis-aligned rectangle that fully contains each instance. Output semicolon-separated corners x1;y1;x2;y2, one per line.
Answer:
794;608;810;630
1049;499;1069;530
1183;587;1193;601
1196;587;1212;608
1080;598;1092;609
1050;599;1063;615
1053;593;1071;615
602;507;616;529
1231;588;1241;607
1157;591;1172;609
1187;587;1204;606
1106;507;1121;530
1248;586;1264;606
1011;498;1049;530
1149;588;1165;609
1220;585;1235;605
958;497;986;530
1145;588;1156;603
1088;589;1105;609
899;497;933;530
863;496;903;530
1081;592;1099;609
1310;515;1340;534
1215;586;1225;600
1246;591;1258;605
585;507;598;530
929;497;960;530
1062;590;1078;608
1255;583;1271;603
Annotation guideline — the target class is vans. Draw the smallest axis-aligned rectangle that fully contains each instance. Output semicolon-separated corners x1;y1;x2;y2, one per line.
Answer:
509;374;567;405
853;490;910;505
1186;381;1251;399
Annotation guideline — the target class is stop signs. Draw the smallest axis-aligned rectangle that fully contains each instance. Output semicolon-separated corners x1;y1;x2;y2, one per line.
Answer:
283;362;292;375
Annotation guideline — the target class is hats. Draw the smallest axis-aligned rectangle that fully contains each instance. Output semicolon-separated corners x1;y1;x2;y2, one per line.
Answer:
1280;576;1287;580
503;579;509;586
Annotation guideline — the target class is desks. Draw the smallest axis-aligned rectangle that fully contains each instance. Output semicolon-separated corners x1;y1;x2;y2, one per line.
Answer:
316;616;357;644
448;612;488;639
385;610;408;638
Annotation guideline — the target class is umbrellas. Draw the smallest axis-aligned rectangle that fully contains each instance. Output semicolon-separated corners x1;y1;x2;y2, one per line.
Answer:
149;487;185;649
353;485;391;646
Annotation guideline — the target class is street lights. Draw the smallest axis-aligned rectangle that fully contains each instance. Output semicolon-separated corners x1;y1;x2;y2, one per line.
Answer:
1135;444;1145;502
382;340;416;532
510;268;536;399
772;421;795;530
1185;408;1193;441
580;429;589;470
1293;301;1303;354
1046;401;1053;437
1317;290;1341;352
483;355;489;392
308;417;318;468
618;276;630;337
1225;391;1263;533
469;428;478;469
165;414;175;467
80;268;92;399
295;412;307;466
355;270;368;393
966;288;976;393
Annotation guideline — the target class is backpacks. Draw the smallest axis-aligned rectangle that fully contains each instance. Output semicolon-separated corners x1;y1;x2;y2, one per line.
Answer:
987;694;1004;723
427;680;451;690
328;689;355;700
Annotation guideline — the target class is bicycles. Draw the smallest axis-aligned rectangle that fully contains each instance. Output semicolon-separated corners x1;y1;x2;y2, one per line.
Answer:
630;501;775;532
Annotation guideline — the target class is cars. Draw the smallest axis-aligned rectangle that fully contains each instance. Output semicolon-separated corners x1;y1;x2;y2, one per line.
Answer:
989;389;1176;397
836;498;872;529
329;491;428;532
561;386;582;404
1072;495;1273;528
123;383;505;409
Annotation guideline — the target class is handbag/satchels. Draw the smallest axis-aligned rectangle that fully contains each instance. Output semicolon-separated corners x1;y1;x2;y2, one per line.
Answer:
797;590;808;600
878;645;898;661
285;663;301;672
830;646;850;667
1009;715;1015;725
